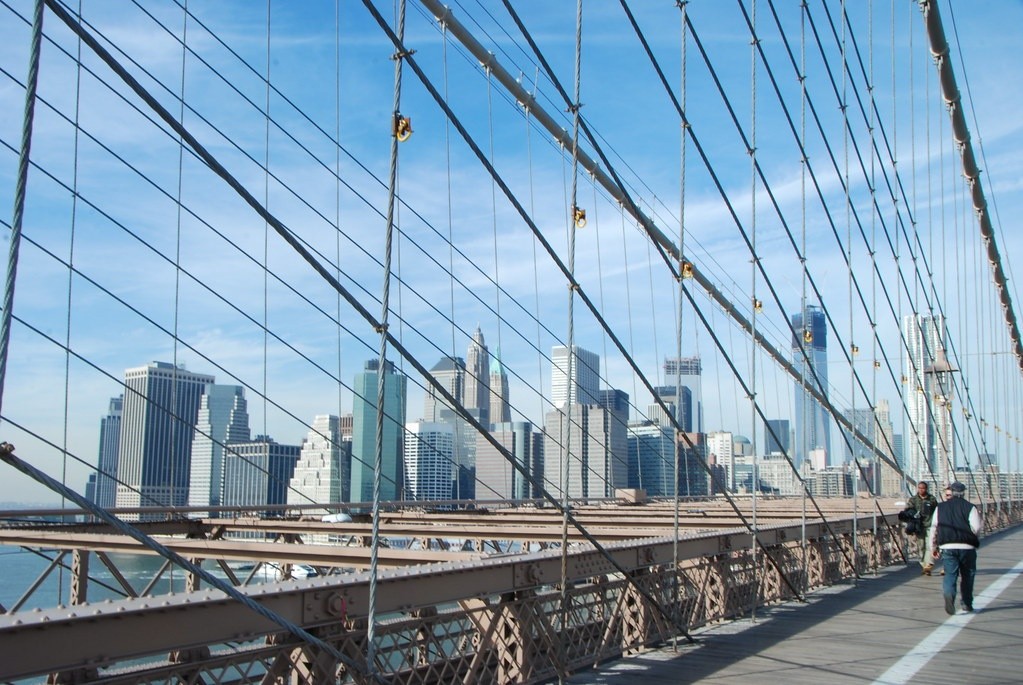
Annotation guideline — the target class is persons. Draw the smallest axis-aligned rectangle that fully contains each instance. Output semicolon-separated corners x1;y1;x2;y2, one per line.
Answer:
898;481;980;615
